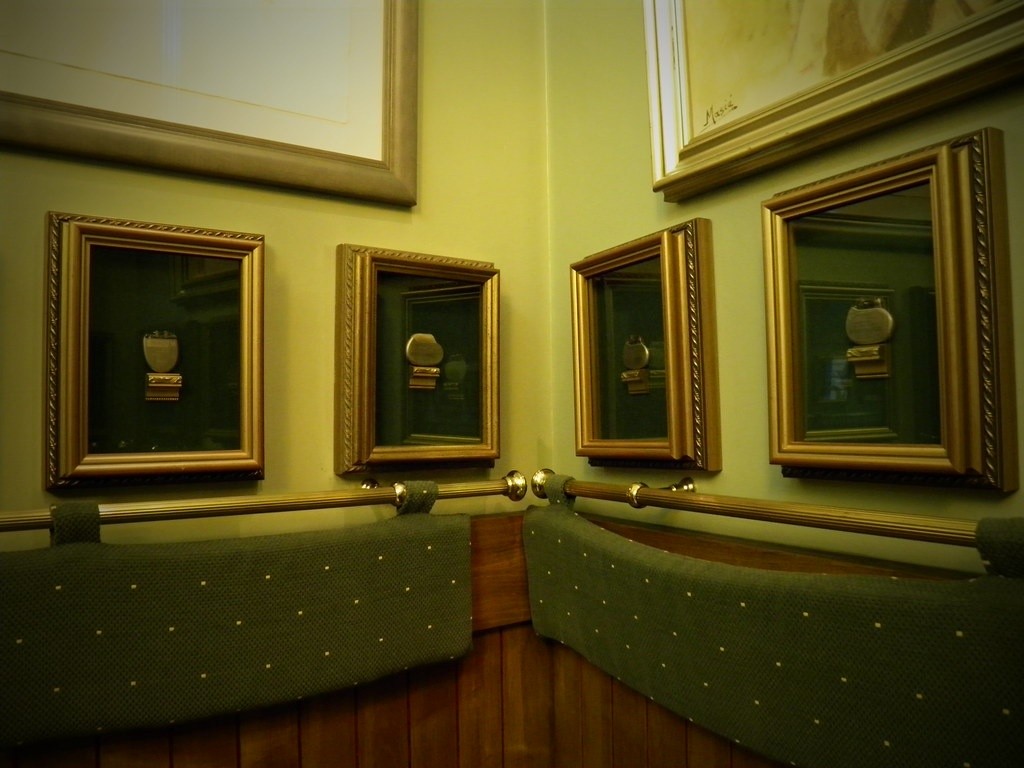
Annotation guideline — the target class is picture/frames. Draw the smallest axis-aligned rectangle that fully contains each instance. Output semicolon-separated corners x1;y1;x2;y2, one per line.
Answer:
0;1;421;205
335;243;501;474
644;0;1024;203
46;210;266;488
761;126;1020;497
570;216;722;473
